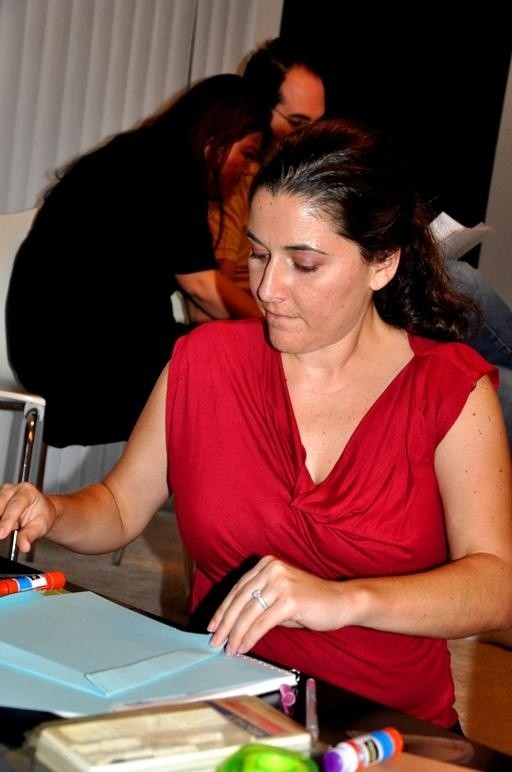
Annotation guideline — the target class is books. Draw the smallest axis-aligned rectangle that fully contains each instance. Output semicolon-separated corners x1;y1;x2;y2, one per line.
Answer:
418;209;495;268
1;585;298;720
30;692;313;772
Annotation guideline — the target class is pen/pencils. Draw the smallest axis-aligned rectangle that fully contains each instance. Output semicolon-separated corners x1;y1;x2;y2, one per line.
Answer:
0;569;67;597
323;726;404;772
306;679;319;743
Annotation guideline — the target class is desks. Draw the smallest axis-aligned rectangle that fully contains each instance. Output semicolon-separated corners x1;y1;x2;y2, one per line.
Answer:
0;553;512;772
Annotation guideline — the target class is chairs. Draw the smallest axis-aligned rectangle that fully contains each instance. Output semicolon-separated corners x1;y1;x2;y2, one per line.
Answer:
0;204;127;496
0;391;48;564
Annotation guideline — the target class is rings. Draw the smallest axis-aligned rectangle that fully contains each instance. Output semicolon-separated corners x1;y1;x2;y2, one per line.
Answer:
252;589;270;613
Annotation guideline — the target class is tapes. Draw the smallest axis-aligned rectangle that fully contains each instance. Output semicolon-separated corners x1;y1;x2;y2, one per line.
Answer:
213;742;315;772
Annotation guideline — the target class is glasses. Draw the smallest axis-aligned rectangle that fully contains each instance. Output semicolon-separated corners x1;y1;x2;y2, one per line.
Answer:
273;106;304;127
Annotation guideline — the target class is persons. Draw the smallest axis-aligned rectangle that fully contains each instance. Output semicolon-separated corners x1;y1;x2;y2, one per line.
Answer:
2;73;276;449
205;35;512;436
0;115;511;739
